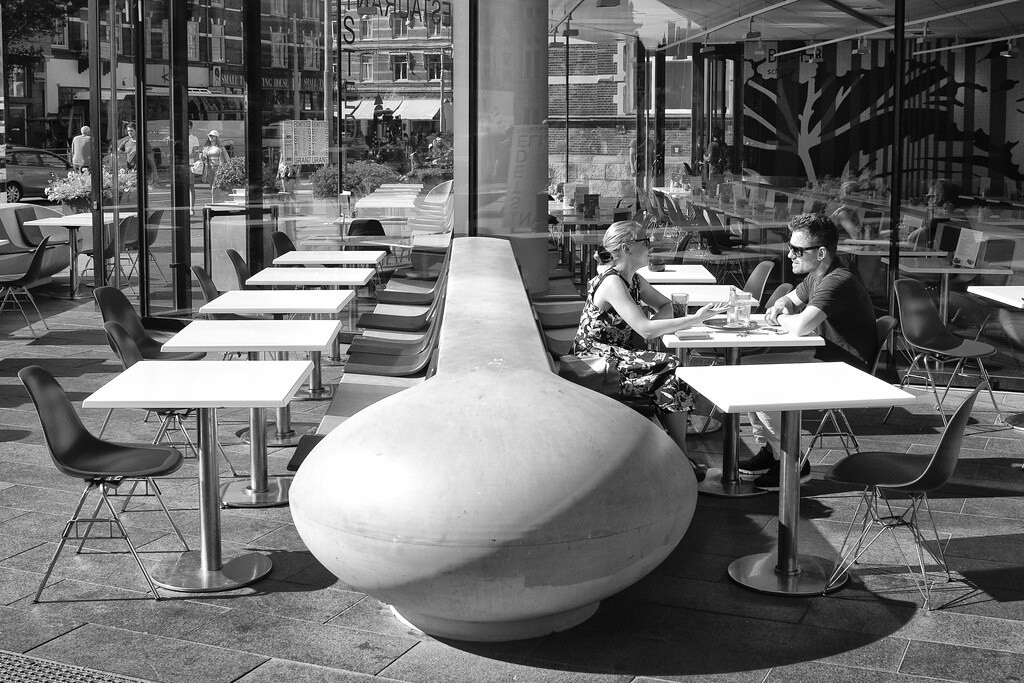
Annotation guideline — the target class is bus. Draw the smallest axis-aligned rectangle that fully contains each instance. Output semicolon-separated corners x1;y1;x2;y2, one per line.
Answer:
67;91;246;167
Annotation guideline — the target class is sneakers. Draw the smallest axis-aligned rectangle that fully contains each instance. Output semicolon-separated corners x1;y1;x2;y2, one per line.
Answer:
737;442;776;475
753;453;811;492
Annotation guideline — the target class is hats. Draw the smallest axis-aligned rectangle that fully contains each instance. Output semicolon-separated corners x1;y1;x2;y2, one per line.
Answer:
208;130;219;138
81;126;91;133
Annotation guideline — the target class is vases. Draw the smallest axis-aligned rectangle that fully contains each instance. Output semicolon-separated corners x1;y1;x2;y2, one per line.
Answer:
61;200;120;216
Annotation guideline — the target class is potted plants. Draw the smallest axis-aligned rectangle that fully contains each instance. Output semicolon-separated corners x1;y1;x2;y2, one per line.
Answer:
213;157;276;202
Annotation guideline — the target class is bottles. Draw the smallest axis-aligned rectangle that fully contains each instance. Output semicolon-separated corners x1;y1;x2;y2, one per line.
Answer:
727;287;738;327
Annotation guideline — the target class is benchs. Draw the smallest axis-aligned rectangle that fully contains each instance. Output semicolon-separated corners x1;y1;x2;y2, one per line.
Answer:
289;235;695;640
408;180;454;251
0;204;84;298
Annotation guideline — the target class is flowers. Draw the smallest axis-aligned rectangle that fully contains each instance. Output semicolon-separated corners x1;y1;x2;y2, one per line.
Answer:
44;165;137;202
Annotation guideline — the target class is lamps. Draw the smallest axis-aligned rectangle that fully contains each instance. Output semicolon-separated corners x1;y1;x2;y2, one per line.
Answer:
548;1;1016;63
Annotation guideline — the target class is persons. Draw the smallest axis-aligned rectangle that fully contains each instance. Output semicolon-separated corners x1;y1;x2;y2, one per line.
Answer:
119;122;157;183
70;127;91;171
188;121;200;215
829;182;882;267
628;122;655;187
703;127;729;179
201;129;230;189
907;178;981;293
275;151;301;214
737;214;878;491
568;220;732;481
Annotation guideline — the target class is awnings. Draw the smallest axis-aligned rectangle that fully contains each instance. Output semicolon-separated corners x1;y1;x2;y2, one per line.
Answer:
333;99;447;121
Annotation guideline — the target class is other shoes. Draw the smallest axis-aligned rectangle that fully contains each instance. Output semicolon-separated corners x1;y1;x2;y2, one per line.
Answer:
688;457;707;481
189;210;194;215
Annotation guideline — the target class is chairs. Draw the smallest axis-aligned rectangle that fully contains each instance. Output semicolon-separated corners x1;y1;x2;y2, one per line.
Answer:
0;216;292;601
345;220;391;288
635;194;1004;612
125;210;167;285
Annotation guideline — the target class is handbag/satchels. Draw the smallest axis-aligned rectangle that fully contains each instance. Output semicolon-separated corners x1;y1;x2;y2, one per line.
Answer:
559;352;623;401
190;159;204;175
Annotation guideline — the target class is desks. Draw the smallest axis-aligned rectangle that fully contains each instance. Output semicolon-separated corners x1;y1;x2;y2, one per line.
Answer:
83;250;387;509
653;178;1024;330
332;183;424;262
23;218;113;301
549;198;917;594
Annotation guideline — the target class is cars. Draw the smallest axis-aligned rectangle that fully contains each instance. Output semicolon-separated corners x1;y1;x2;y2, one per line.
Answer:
5;147;72;203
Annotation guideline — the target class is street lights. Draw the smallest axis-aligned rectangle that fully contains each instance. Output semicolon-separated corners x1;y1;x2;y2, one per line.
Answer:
561;29;580;183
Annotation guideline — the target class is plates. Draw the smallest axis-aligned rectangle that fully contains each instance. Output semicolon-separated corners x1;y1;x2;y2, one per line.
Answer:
703;319;757;330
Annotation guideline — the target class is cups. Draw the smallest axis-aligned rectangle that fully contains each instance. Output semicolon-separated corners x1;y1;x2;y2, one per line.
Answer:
737;301;751;326
780;210;788;220
671;293;689;319
806;182;814;191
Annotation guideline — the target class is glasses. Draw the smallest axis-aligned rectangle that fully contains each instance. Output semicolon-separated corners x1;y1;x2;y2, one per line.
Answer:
925;194;936;201
620;238;650;249
787;243;827;257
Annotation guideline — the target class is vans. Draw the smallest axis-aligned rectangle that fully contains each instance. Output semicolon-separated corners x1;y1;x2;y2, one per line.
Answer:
264;123;320;178
